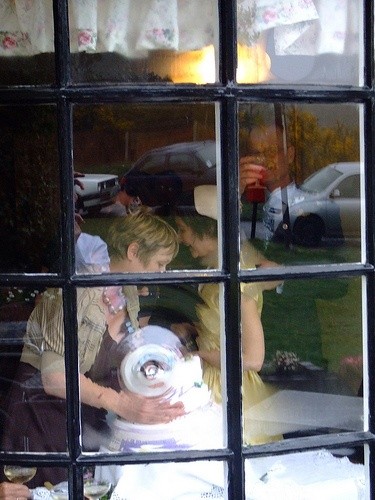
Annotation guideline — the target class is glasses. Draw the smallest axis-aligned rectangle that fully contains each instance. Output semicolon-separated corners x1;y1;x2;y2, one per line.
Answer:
242;143;293;157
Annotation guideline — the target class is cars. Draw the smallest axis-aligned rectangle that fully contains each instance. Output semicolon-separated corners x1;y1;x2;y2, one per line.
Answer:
73;172;121;216
262;161;360;251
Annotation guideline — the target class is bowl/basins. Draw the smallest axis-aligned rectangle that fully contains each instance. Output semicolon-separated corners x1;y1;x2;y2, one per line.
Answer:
50;481;69;500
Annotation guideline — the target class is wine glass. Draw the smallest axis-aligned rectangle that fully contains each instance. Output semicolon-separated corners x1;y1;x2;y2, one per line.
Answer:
84;480;111;500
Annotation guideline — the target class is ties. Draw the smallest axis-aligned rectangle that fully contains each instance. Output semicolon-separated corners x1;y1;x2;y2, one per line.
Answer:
262;192;274;252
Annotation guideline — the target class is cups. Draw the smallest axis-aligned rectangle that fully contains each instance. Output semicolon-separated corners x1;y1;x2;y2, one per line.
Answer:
4;465;37;484
244;156;270;201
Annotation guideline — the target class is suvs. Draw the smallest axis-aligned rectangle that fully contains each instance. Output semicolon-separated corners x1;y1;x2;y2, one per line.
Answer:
110;139;216;209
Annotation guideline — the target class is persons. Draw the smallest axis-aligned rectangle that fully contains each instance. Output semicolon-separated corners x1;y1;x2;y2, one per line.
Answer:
0;482;31;500
239;115;349;392
19;212;185;485
95;171;153;218
174;190;284;444
